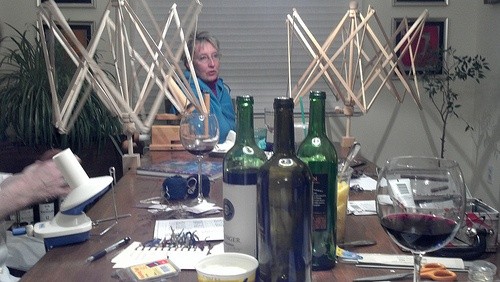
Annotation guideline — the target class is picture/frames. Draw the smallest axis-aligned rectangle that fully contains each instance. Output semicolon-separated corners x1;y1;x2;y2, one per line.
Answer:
389;16;449;81
36;0;97;9
36;20;95;62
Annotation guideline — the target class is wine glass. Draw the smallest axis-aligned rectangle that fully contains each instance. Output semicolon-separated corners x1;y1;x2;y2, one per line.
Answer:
375;156;466;282
179;114;220;207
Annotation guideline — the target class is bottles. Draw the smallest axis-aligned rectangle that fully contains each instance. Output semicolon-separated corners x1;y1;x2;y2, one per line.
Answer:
222;95;268;259
296;91;336;271
257;96;312;282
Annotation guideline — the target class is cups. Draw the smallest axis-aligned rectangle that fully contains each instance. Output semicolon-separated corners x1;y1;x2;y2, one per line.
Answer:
336;164;354;249
294;121;310;146
262;108;274;135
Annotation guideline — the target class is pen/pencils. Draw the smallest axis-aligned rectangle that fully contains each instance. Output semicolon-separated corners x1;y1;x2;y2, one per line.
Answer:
84;235;132;265
99;221;118;236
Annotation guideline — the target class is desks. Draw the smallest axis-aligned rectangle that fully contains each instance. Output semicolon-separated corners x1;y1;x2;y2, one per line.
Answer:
17;150;470;282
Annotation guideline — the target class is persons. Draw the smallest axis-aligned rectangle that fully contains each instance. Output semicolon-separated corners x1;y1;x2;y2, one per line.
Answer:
168;31;235;143
0;149;71;282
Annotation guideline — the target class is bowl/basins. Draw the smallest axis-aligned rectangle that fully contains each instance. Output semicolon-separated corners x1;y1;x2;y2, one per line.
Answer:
194;252;259;282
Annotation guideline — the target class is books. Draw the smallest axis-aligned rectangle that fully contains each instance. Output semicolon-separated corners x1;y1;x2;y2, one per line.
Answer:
136;159;224;181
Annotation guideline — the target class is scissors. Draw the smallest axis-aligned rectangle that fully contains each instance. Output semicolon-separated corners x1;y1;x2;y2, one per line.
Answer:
350;261;458;281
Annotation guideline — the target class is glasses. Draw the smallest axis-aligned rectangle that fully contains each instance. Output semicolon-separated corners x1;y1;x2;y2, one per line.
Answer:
198;53;223;62
